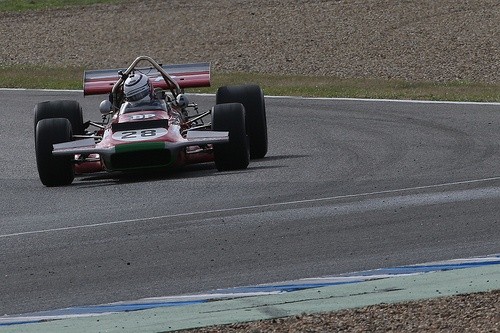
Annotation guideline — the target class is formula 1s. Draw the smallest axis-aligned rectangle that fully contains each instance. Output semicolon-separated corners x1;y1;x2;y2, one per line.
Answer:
33;55;268;188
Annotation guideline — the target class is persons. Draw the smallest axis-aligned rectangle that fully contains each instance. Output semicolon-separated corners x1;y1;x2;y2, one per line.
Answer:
122;74;172;102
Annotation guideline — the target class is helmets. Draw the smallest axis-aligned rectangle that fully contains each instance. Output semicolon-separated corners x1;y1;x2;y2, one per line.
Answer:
124;73;152;102
